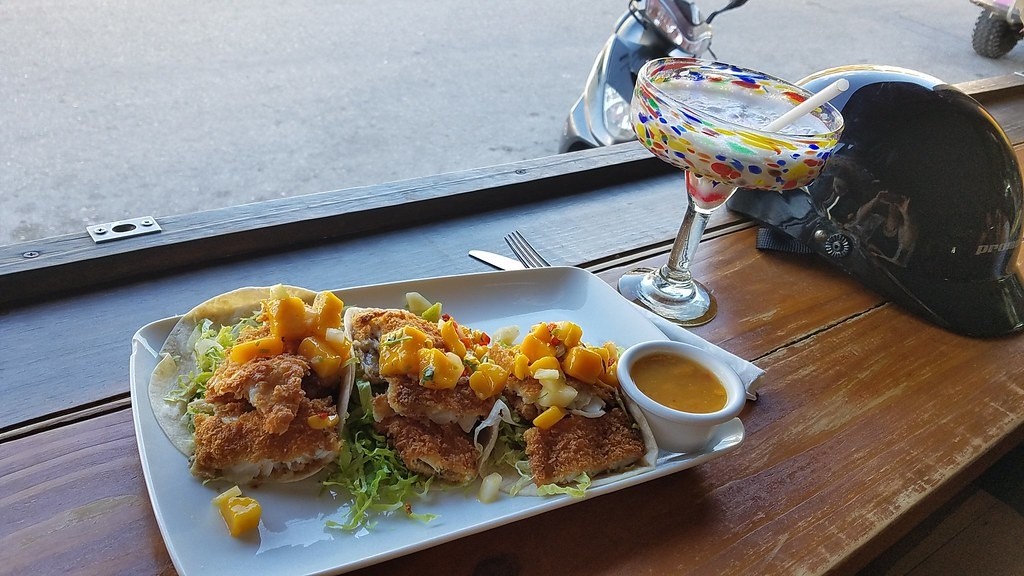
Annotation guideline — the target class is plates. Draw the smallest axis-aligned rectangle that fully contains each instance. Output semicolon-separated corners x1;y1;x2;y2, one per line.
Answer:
128;264;746;576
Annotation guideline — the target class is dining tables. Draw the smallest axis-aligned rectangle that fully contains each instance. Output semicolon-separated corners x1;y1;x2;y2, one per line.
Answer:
2;68;1024;574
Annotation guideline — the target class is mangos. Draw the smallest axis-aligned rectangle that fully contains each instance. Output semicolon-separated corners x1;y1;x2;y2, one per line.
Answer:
228;283;618;426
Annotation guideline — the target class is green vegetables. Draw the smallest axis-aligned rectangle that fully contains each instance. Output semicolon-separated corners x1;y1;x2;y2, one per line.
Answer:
323;384;594;533
163;305;265;450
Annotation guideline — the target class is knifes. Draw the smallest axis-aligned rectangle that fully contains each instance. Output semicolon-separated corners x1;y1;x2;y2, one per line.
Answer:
468;249;536;272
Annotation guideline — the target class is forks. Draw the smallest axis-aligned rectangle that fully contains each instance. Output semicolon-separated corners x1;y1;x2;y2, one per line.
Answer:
504;228;550;272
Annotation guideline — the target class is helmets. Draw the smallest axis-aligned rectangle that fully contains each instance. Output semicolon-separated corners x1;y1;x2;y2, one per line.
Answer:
723;65;1023;339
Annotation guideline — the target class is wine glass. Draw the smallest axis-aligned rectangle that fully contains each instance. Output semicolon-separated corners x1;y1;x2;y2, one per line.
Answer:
615;55;845;330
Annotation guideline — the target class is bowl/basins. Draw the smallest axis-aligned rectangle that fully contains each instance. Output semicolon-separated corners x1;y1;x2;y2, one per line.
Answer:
614;339;746;456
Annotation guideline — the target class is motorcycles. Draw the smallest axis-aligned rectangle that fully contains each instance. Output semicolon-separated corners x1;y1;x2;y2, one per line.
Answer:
559;1;752;160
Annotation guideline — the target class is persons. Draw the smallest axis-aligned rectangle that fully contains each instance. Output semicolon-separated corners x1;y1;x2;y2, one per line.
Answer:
855;189;917;268
